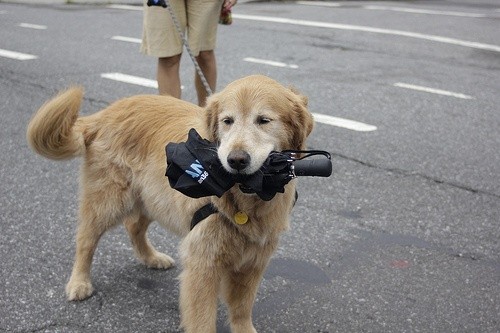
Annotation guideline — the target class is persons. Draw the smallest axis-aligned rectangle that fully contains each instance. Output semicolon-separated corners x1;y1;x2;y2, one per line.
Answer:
142;0;237;108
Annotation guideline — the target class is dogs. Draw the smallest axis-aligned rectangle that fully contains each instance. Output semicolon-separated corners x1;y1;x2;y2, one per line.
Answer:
26;73;315;331
